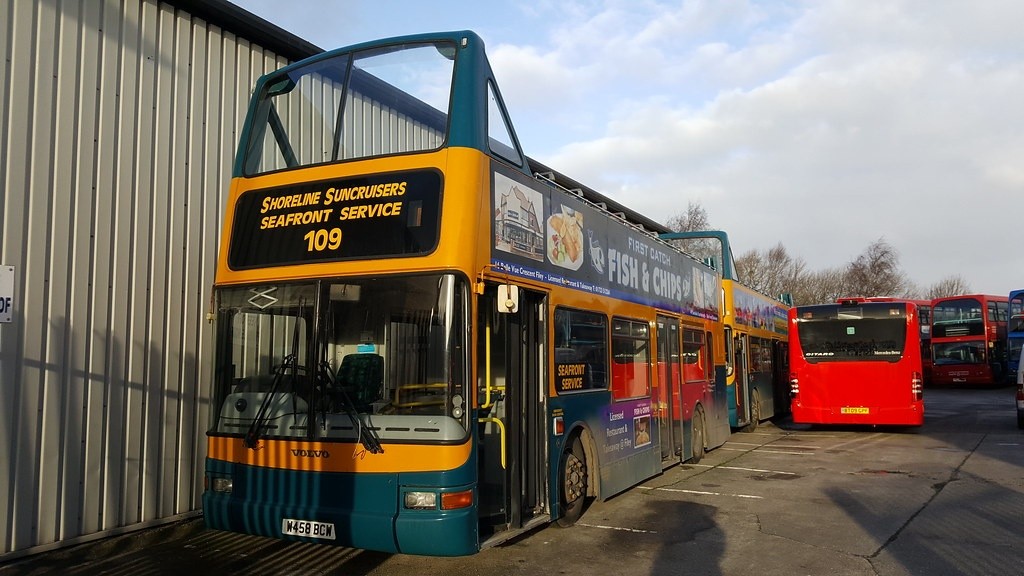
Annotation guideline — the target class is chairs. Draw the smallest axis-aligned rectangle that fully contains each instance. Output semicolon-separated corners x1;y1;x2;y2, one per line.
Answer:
329;354;381;411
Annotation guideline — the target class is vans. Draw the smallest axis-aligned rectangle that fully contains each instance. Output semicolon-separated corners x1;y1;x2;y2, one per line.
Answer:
1016;344;1024;428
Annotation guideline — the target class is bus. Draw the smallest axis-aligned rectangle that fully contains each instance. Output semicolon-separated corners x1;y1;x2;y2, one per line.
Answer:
1006;289;1024;378
202;30;730;557
655;232;791;433
866;298;987;379
930;295;1021;388
786;298;924;430
